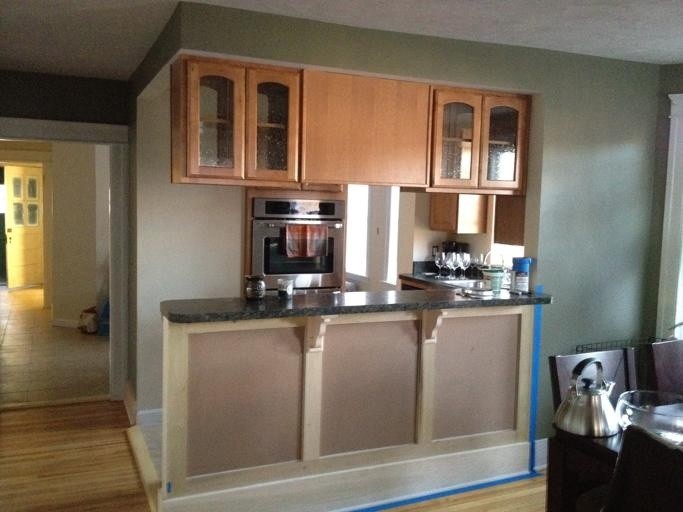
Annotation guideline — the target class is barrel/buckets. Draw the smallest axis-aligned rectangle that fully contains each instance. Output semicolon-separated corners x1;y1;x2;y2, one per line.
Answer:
481;249;505;291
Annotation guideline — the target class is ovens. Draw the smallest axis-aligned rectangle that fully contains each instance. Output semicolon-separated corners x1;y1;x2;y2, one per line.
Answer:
250;197;345;291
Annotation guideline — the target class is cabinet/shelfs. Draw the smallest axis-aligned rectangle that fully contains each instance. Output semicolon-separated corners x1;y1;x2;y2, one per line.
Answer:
303;69;433;188
170;55;302;190
400;85;532;199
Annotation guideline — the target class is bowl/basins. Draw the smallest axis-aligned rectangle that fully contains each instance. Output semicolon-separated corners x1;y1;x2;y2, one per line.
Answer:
614;388;683;449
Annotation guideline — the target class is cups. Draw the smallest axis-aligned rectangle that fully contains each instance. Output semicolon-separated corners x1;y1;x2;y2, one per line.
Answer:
277;279;293;299
490;274;501;293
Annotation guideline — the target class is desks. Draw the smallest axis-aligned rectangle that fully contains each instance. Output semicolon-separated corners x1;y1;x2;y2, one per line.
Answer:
548;402;683;512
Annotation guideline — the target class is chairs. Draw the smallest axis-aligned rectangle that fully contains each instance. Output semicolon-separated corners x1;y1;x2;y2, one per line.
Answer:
549;338;683;414
600;425;683;512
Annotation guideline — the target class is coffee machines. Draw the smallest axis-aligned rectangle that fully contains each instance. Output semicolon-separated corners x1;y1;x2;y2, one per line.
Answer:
432;241;469;277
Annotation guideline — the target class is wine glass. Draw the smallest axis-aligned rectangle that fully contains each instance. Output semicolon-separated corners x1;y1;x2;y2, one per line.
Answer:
434;252;470;280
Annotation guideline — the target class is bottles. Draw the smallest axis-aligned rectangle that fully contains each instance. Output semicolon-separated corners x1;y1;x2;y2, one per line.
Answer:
510;257;531;293
245;275;265;300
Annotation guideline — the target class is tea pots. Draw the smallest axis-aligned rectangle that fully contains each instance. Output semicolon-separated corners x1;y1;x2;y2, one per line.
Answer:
553;357;620;436
